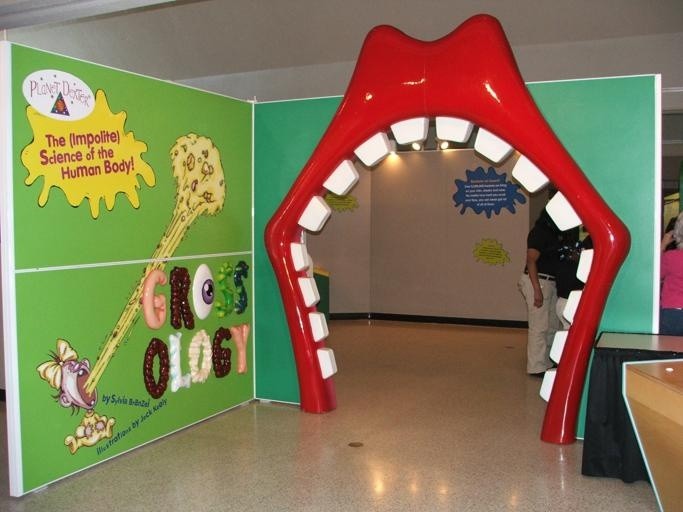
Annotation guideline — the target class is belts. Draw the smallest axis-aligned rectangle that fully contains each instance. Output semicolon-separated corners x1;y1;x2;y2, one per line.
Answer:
523;271;557;280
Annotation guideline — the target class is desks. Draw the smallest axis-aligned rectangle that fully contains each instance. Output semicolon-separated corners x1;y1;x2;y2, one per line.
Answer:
595;330;682;512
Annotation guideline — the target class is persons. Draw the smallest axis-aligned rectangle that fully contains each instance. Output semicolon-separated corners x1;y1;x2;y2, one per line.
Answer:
517;209;561;378
661;212;683;335
